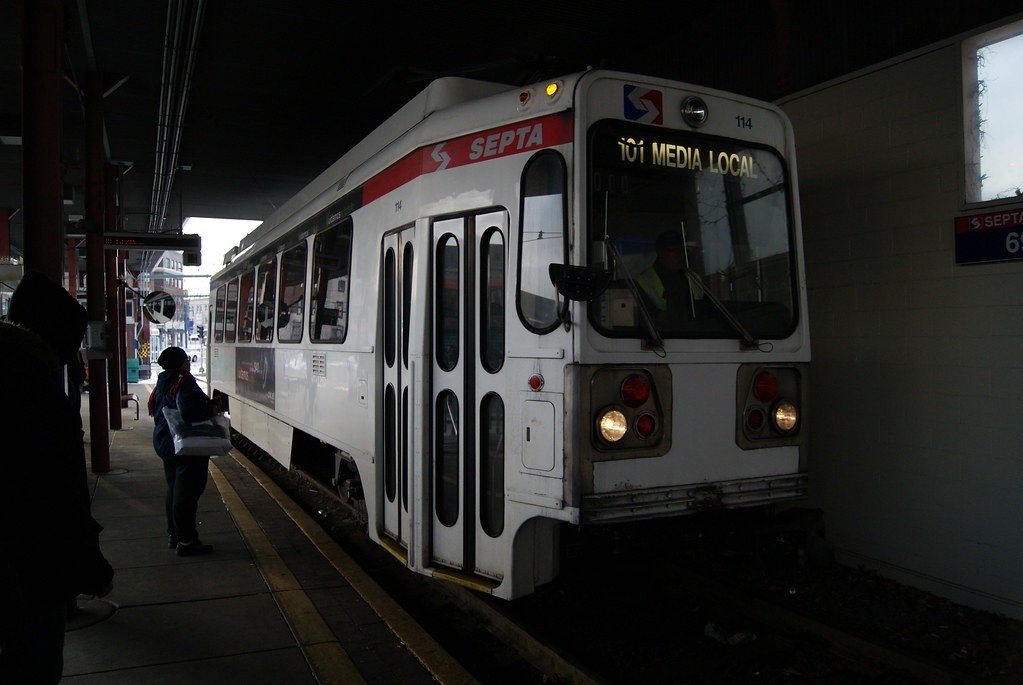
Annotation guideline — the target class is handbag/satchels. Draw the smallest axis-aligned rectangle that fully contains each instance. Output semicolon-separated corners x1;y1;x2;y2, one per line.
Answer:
162;375;233;456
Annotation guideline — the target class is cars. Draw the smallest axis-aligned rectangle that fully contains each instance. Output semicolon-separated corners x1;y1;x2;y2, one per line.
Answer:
159;345;198;362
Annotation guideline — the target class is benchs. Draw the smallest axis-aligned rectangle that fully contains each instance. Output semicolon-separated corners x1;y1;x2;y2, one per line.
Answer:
120;393;139;419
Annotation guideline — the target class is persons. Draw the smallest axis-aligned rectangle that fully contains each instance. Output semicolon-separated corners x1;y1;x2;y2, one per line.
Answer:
0;271;114;685
640;231;718;321
148;347;223;557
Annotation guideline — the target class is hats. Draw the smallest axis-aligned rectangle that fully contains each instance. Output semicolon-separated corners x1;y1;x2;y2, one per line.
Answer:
158;347;187;371
656;231;687;250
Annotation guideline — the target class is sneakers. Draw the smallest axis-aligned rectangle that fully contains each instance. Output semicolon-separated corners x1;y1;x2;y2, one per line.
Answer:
167;535;178;548
176;540;213;557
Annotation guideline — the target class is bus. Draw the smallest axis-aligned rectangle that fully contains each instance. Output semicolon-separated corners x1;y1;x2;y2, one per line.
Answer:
205;70;814;603
146;293;175;323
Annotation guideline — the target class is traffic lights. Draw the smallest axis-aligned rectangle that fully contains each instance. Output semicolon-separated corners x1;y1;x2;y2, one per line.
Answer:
197;326;204;338
